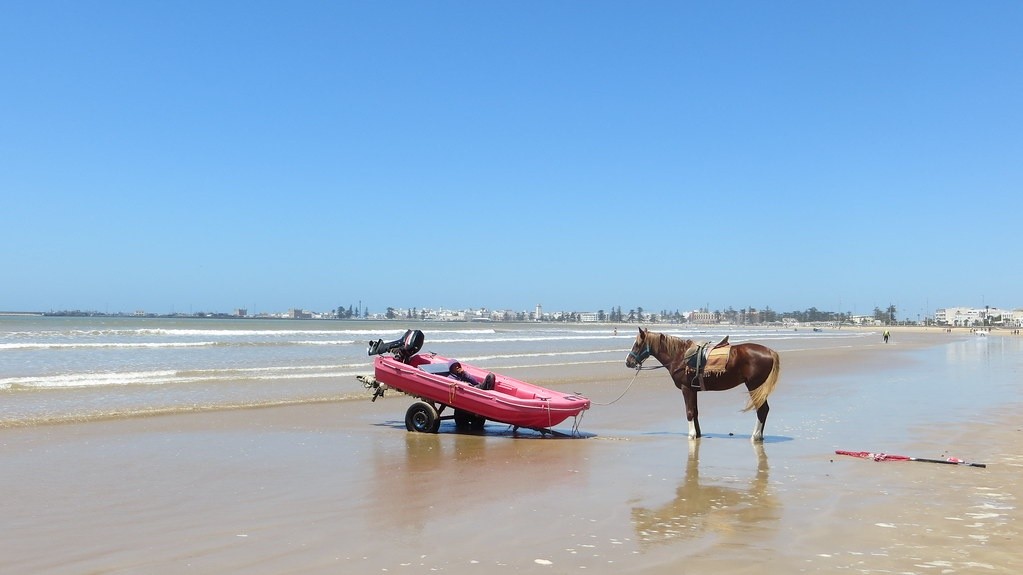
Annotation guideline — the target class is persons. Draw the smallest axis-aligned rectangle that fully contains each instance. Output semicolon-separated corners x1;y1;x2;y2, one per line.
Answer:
970;326;1019;336
448;359;496;390
883;329;890;343
947;328;951;334
614;327;617;335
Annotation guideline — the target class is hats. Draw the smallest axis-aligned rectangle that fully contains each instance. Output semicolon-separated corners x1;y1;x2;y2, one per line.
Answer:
447;359;462;372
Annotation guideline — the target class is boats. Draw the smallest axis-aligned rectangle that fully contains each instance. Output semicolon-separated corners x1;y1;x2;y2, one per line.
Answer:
359;330;590;430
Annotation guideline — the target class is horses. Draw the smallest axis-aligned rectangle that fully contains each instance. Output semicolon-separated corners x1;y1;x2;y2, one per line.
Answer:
625;327;780;442
